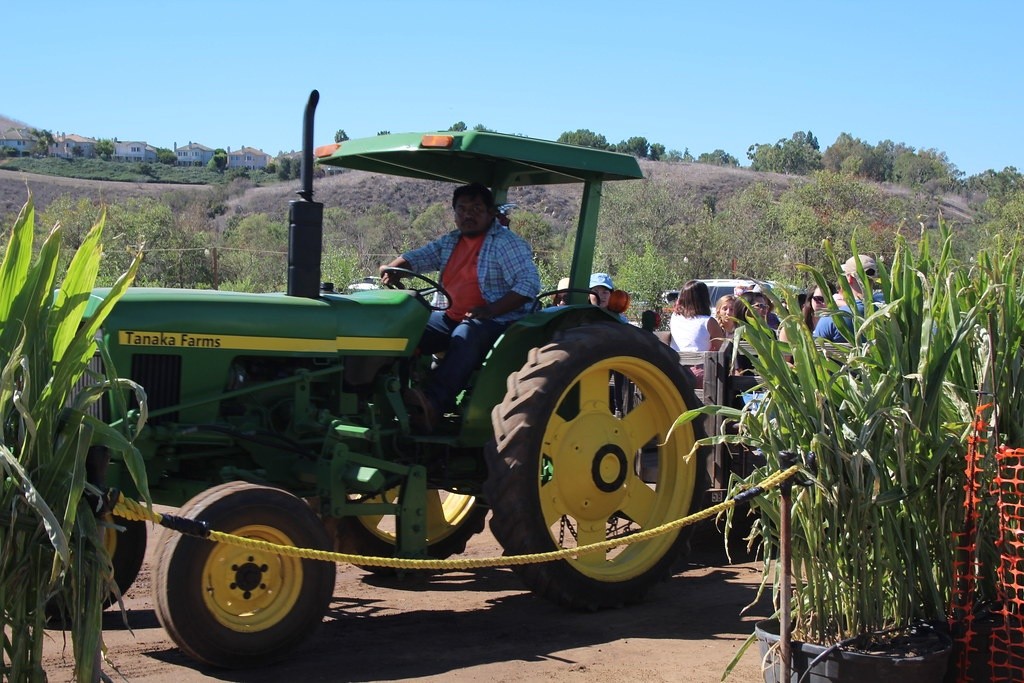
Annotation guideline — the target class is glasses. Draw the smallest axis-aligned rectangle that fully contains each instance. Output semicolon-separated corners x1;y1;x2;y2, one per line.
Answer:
455;206;488;216
812;295;824;303
595;291;610;296
750;303;770;311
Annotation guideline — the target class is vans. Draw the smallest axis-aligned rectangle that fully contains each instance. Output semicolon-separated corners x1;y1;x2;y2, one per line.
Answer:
692;278;779;318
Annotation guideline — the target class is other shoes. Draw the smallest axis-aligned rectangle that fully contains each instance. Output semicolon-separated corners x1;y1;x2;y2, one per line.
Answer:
403;387;440;437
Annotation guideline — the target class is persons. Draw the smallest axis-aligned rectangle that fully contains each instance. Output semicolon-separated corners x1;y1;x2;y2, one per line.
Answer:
380;183;541;435
587;273;628;324
550;278;570;306
669;254;885;389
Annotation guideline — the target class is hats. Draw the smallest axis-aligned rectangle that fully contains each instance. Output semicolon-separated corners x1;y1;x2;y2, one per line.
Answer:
744;282;771;293
838;254;878;280
589;272;613;289
557;277;571;290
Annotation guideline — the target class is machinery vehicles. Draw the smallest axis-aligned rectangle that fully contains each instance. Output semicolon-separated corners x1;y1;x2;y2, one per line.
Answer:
45;87;712;673
